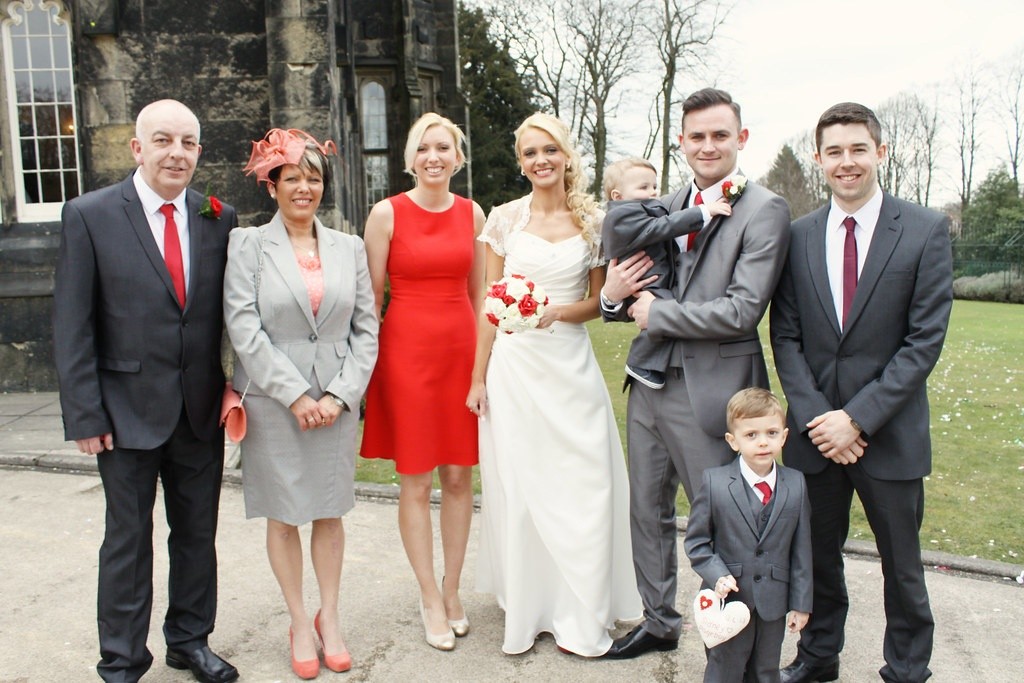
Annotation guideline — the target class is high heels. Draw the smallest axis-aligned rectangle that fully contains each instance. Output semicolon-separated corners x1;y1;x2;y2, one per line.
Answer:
289;618;320;679
441;573;469;636
420;597;456;651
314;609;350;673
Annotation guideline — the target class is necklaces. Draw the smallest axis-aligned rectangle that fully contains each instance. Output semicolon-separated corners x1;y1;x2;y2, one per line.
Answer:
288;236;319;258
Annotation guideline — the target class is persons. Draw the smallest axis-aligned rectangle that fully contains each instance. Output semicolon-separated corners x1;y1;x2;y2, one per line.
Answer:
464;112;645;658
770;101;954;683
223;128;378;680
51;97;239;683
364;112;487;652
599;87;791;658
602;159;731;389
684;387;812;683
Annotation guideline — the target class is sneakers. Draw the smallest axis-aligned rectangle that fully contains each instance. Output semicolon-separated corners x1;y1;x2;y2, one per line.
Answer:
626;363;665;390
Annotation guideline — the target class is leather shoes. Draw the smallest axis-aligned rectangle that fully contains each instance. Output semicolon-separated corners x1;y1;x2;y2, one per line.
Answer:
166;647;239;683
605;625;678;659
779;655;840;683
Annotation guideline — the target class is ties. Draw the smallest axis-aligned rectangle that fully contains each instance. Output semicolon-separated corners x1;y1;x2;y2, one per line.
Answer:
755;482;773;505
842;216;859;330
687;192;703;251
160;203;186;310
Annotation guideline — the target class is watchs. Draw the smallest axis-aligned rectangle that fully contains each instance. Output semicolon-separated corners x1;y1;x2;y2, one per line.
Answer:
843;408;864;435
326;392;350;412
602;292;622;307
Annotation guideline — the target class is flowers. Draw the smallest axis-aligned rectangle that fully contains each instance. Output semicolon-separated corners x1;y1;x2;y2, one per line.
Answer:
484;272;557;335
721;174;748;200
198;183;223;222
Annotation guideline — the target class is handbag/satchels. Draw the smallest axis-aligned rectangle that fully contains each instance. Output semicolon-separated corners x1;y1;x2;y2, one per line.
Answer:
218;381;247;443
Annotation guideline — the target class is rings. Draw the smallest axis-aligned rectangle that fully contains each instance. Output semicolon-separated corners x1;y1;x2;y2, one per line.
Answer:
306;417;314;422
322;417;325;426
469;406;477;412
